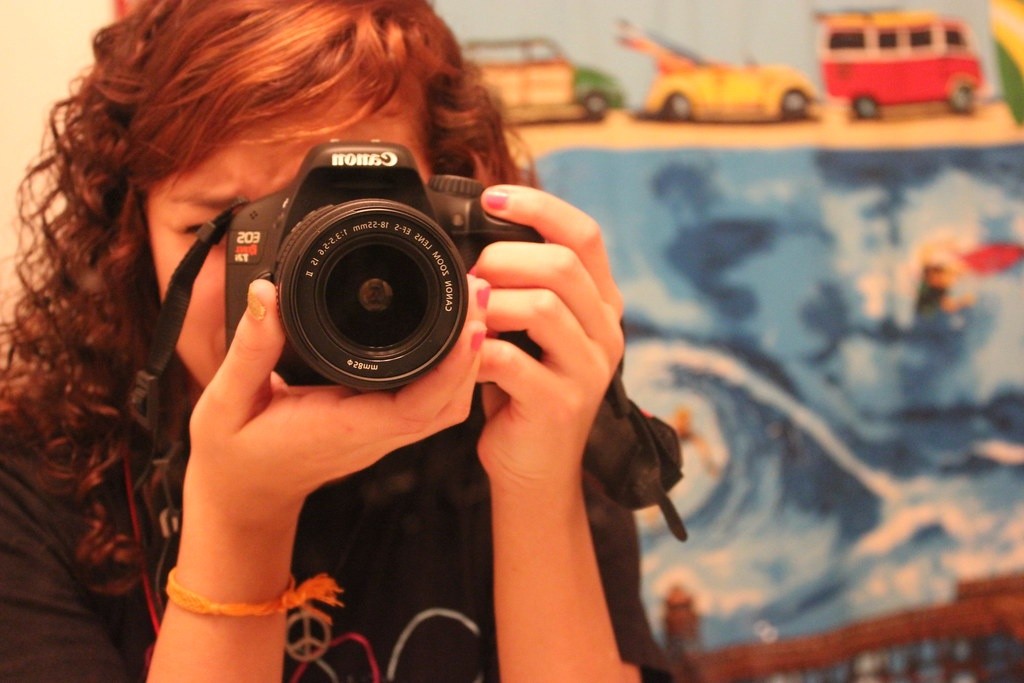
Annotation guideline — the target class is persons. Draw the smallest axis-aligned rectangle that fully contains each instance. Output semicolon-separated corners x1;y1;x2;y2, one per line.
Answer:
0;0;679;683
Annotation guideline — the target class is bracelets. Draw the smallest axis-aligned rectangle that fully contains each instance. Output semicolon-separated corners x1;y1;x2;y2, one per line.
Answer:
164;565;346;625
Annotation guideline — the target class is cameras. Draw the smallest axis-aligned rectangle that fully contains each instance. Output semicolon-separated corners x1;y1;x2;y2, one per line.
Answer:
222;140;546;392
